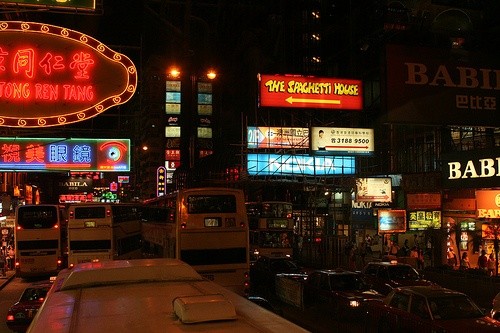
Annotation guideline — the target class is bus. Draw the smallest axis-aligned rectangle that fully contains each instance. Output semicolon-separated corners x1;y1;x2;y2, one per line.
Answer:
24;257;308;333
245;200;293;262
68;201;143;269
13;204;68;278
141;187;252;299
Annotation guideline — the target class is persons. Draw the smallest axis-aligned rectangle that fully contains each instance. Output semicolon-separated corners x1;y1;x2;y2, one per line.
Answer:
264;230;495;275
316;130;327;151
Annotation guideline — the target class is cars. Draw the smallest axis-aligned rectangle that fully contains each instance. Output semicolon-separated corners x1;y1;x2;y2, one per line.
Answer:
247;260;500;333
6;275;56;333
